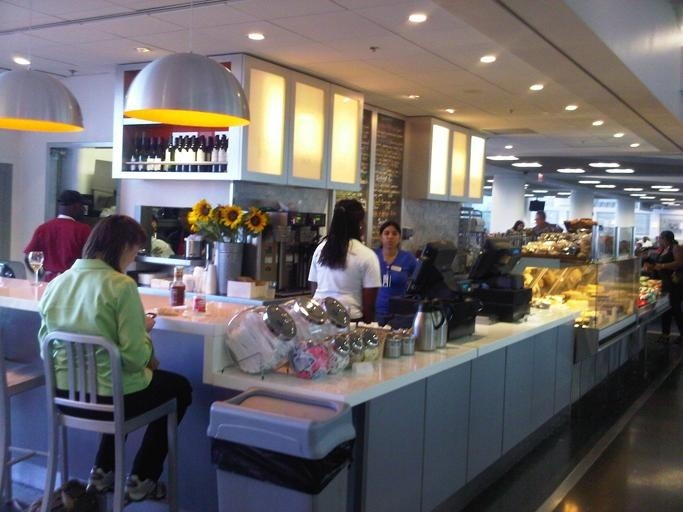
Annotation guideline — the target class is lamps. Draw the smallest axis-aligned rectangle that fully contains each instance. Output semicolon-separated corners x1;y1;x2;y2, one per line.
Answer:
122;2;252;129
1;28;85;132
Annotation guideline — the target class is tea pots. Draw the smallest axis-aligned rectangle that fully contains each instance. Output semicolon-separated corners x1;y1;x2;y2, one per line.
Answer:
412;298;455;351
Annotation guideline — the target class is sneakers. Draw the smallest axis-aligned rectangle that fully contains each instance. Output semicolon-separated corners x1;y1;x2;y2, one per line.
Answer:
87;465;114;495
124;474;166;501
657;334;681;344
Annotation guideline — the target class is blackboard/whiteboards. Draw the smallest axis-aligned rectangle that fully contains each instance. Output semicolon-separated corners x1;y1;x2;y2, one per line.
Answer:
327;102;410;252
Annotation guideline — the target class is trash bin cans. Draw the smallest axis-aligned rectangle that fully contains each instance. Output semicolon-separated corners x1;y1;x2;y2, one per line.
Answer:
207;385;357;512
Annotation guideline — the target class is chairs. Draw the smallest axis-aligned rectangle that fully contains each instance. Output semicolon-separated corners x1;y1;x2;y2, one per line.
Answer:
1;341;69;512
39;330;178;512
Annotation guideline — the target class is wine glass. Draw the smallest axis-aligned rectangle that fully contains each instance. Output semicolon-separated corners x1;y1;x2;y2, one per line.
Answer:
28;251;44;288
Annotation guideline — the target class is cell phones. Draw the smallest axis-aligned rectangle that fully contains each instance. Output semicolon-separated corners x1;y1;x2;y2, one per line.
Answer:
145;313;156;318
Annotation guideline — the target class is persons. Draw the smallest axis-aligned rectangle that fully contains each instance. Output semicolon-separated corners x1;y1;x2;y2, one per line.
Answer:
531;210;563;235
37;213;194;503
635;230;683;344
167;210;194;257
24;189;92;281
373;221;418;324
307;198;383;324
151;215;174;256
511;220;525;231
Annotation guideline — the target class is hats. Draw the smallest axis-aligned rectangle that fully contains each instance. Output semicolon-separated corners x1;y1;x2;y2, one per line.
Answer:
638;246;648;252
58;189;91;205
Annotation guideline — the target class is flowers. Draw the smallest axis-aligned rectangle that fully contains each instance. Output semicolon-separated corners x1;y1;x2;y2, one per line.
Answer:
186;196;270;242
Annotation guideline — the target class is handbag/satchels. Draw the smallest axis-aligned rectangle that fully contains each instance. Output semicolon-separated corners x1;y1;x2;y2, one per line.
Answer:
671;271;683;283
27;478;98;511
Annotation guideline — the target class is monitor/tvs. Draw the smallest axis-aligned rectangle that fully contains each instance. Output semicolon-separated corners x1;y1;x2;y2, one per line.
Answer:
468;237;522;282
406;240;460;296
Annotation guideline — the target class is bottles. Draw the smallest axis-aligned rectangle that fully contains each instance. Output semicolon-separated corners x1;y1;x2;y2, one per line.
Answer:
130;132;226;173
171;265;184;305
225;296;378;380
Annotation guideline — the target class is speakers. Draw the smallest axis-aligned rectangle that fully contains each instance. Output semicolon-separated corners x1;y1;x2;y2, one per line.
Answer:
530;200;545;211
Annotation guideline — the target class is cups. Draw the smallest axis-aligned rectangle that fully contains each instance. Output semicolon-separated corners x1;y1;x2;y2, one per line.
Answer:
185;263;216;294
192;295;207;312
385;337;415;358
